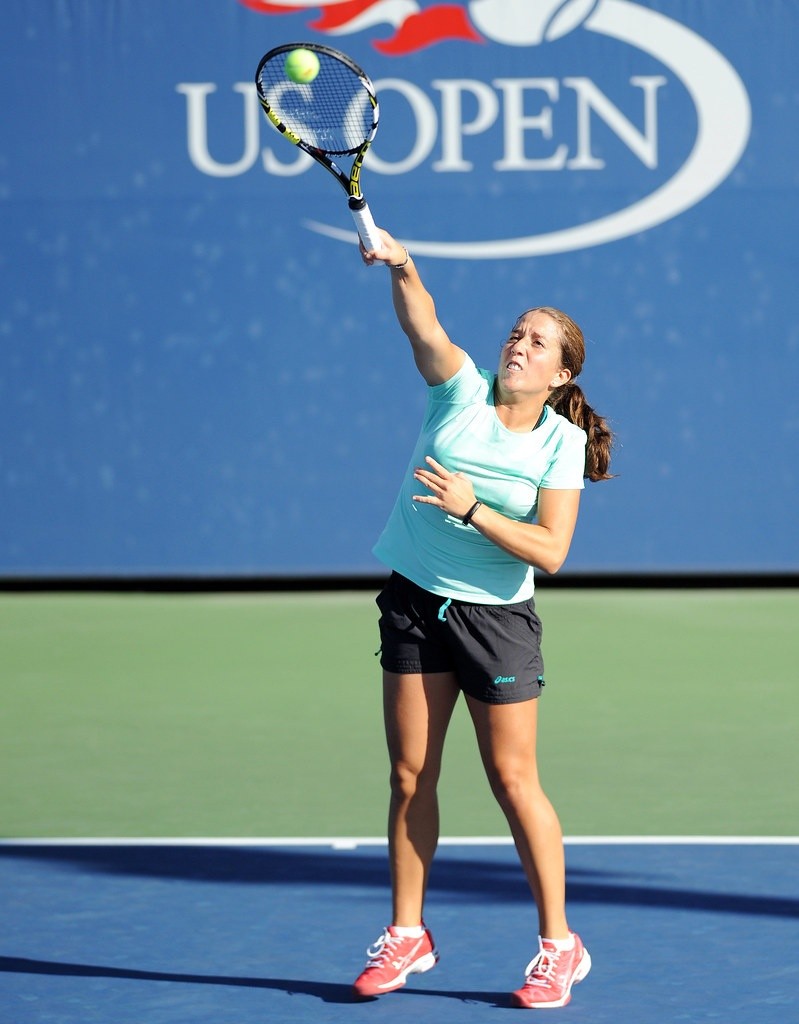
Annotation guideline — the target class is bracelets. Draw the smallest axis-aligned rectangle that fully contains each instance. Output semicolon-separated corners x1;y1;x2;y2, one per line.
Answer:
385;246;409;269
462;500;482;526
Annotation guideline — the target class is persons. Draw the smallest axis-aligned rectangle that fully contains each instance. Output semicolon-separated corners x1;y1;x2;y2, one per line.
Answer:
354;228;612;1010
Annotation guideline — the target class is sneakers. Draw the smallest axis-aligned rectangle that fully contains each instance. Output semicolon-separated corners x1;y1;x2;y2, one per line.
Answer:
510;928;592;1008
352;918;440;997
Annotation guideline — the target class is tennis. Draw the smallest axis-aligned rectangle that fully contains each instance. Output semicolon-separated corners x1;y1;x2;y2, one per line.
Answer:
285;49;320;85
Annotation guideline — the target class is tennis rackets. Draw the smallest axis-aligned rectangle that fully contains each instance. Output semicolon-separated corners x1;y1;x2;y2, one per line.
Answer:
255;43;388;266
